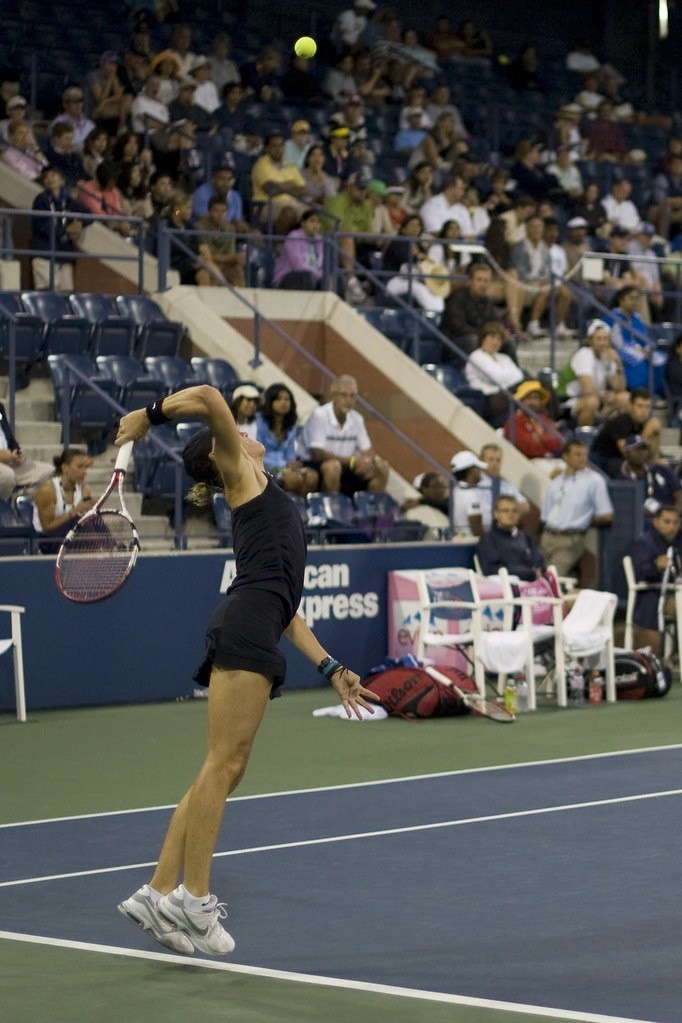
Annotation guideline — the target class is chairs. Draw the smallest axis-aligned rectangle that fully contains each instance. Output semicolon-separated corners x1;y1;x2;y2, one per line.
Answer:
0;0;682;214
0;604;27;722
387;556;682;716
0;290;505;548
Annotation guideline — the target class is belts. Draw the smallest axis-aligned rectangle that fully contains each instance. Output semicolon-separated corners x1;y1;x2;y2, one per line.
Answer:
543;523;588;538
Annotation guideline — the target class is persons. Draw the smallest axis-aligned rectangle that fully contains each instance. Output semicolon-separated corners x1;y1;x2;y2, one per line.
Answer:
114;385;380;957
0;0;682;654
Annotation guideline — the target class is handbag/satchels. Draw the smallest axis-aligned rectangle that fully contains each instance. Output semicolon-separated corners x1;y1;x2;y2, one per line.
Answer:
582;650;669;702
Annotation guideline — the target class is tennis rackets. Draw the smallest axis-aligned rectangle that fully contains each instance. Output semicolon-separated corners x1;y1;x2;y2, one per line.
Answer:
55;439;141;604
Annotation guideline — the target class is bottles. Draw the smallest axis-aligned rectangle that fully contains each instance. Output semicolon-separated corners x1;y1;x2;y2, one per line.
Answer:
514;674;529;713
571;668;585;707
504;679;518;716
588;669;604;704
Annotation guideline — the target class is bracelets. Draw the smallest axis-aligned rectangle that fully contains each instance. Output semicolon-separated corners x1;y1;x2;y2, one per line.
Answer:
349;457;356;468
146;396;173;427
316;656;348;680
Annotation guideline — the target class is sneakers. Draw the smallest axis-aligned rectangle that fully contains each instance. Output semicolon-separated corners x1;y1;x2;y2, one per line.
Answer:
116;883;196;955
156;884;236;957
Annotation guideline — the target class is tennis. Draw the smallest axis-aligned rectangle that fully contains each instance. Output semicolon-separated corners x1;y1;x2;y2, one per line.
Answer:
295;36;316;60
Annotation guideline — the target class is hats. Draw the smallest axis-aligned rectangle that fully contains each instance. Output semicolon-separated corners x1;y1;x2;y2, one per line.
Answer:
565;215;588;229
367;179;390;197
232;384;262;402
290;121;310;133
61;87;86;103
151;51;180;76
6;95;27;109
189;55;212;75
419;258;453;297
450;450;489;474
512;380;550;407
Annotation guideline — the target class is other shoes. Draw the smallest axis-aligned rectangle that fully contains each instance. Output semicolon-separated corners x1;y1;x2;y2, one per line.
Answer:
527;323;547;339
347;276;366;302
557;325;578;338
